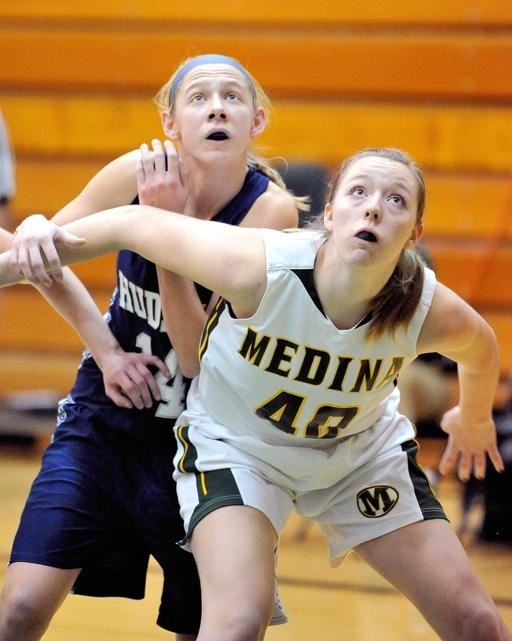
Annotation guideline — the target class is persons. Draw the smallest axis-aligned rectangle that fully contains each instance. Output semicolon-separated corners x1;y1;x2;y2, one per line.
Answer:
0;145;512;640
397;353;458;438
453;375;511;549
0;51;309;641
0;228;174;412
